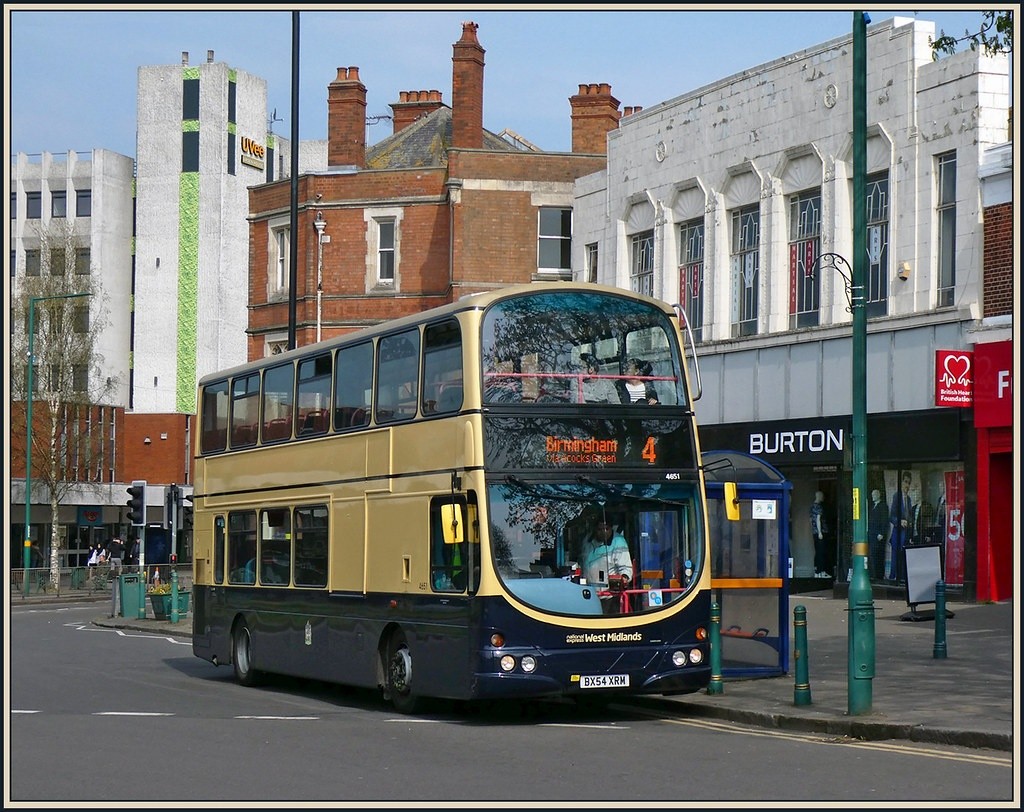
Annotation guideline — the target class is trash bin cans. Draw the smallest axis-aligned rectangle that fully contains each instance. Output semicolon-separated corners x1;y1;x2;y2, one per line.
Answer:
119;574;140;618
72;568;85;589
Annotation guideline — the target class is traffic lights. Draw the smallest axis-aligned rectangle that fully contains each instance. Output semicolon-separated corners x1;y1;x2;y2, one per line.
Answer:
126;480;147;526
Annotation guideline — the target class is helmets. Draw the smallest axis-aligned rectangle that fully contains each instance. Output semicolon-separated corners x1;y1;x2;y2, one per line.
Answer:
579;353;599;371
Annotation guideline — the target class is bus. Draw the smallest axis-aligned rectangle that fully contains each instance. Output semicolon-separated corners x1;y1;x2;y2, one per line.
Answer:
193;281;740;717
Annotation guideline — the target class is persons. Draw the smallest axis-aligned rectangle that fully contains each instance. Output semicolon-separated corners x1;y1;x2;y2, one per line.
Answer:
888;472;912;581
571;353;659;406
575;514;633;614
31;540;46;567
869;490;888;580
809;491;832;578
909;481;945;581
88;537;140;577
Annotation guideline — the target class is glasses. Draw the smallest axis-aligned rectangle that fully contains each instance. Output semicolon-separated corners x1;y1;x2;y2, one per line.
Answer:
626;367;635;371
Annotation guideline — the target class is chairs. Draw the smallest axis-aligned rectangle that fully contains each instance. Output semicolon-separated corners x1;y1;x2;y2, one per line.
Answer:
212;381;461;450
231;532;319;585
484;379;521;404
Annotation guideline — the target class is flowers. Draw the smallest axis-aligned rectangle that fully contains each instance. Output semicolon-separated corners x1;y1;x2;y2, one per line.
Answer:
148;585;181;594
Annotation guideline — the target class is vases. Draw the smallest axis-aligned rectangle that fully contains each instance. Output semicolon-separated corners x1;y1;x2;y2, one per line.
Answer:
149;590;192;620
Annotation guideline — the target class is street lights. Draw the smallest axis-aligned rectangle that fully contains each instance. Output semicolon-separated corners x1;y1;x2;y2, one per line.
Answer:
22;292;94;597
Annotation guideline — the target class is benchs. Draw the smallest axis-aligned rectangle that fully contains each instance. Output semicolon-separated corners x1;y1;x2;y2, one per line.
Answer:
246;537;301;559
